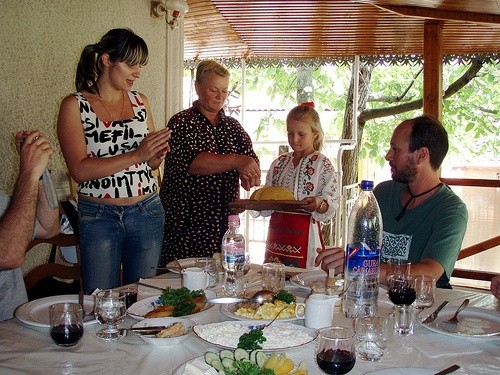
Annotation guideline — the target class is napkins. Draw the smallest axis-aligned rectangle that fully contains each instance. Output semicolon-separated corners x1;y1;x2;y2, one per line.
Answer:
406;334;483;358
138;277;182;297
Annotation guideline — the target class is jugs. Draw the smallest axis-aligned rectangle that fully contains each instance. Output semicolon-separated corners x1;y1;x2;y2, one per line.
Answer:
295;294;342;330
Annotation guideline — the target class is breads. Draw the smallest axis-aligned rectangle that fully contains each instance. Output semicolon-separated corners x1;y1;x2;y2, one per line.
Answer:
212;253;223;272
156;322;184;337
250;186;296;200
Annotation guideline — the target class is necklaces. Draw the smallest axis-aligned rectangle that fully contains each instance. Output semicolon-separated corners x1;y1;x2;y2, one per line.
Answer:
395;182;443;222
96;91;124;121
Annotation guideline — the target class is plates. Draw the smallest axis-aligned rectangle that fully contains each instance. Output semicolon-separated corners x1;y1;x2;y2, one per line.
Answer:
416;306;500;338
173;352;226;375
219;296;305;322
15;295;98;328
192;320;320;354
124;293;218;321
363;368;452;375
264;264;305;276
167;257;209;274
290;273;344;290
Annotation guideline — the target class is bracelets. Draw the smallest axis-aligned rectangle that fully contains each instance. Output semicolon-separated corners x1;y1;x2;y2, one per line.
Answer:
317;200;324;210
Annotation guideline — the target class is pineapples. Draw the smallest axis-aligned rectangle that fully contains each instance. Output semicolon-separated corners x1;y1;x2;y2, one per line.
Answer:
264;353;308;375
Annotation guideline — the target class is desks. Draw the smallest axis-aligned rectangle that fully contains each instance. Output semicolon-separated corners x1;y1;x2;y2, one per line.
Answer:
0;261;500;375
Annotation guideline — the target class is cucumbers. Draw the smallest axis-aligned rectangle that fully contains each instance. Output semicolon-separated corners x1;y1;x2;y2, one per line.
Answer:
204;347;269;372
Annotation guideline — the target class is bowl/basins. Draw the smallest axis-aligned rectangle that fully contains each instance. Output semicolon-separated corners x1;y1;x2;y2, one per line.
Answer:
130;316;196;346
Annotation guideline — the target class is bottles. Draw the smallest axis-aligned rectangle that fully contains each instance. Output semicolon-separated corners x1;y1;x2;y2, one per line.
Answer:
221;215;246;296
341;181;383;319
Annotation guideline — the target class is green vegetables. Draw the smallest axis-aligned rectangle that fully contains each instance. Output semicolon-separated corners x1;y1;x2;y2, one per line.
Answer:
159;286;205;317
238;330;266;350
224;360;276;375
275;290;296;304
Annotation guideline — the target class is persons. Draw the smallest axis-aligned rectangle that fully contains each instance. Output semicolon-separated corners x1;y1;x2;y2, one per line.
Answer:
315;114;468;289
159;61;261;273
231;102;340;269
57;28;173;292
0;130;60;321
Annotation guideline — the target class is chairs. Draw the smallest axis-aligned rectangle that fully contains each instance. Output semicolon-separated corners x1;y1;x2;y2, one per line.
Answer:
22;200;84;299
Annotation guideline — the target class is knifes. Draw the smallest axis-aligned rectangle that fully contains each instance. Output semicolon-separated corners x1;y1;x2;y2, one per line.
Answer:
421;300;449;323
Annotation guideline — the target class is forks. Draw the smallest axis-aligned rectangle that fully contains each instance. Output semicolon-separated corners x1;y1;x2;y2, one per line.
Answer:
442;298;469;324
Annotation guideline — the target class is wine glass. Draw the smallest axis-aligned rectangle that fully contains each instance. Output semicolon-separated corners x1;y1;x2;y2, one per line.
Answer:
93;289;126;343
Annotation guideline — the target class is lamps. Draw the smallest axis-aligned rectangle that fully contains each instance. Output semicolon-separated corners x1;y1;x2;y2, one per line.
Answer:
152;0;189;30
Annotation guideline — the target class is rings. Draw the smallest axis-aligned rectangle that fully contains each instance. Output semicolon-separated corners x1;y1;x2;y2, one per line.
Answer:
247;173;250;176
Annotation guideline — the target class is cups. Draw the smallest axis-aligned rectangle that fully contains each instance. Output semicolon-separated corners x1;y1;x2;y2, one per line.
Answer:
414;277;435;309
315;325;356;375
196;258;220;288
118;287;139;308
261;263;286;295
243;252;250;275
354;315;387;362
386;262;412;275
49;302;85;347
387;273;417;306
394;305;414;336
180;268;210;291
